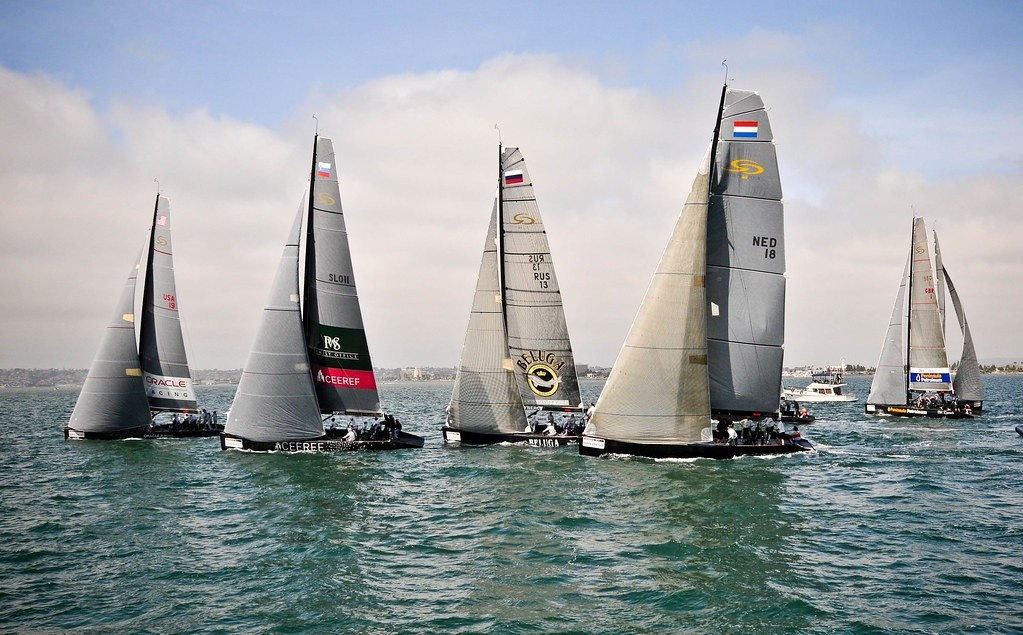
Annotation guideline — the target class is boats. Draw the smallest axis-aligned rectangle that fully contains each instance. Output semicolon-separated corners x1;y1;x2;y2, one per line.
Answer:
782;383;860;405
808;366;844;386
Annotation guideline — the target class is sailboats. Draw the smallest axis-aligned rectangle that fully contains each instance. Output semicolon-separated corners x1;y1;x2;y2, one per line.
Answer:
221;135;427;454
437;141;590;450
59;194;226;442
580;88;817;464
860;219;986;420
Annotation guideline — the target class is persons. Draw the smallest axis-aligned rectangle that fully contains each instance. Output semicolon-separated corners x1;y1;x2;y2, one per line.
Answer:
171;409;217;430
330;414;401;441
530;403;595;435
718;416;800;444
